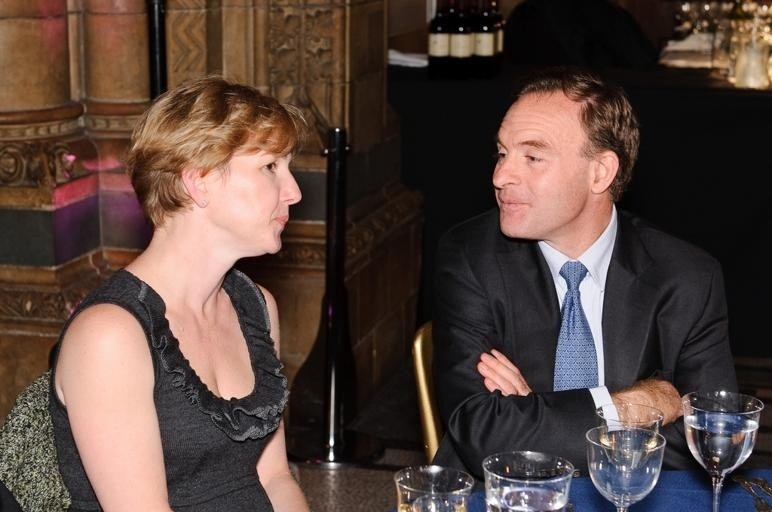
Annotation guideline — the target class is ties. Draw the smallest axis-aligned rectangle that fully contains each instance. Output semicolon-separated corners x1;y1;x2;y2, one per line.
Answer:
553;261;599;392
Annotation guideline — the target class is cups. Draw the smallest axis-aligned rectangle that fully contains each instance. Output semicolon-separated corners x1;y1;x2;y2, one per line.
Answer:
481;450;574;512
585;404;667;511
393;464;475;511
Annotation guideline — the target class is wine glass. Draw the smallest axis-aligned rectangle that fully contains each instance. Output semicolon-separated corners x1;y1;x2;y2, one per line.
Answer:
682;390;765;511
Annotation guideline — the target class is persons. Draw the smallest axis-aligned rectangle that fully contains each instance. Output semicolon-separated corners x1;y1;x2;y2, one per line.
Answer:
426;71;741;481
48;77;309;512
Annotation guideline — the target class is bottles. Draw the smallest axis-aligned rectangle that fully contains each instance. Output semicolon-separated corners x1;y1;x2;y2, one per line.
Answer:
427;0;505;61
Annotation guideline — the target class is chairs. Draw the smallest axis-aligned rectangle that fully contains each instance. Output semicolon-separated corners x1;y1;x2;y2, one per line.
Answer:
407;323;447;477
1;366;75;512
